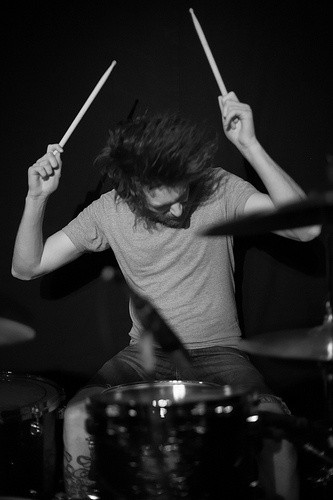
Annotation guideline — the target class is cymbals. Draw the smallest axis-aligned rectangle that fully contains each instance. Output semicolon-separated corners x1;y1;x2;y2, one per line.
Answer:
237;319;333;361
200;189;332;237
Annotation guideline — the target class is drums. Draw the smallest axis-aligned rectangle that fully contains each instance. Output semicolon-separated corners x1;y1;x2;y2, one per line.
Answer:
0;367;64;499
85;379;270;500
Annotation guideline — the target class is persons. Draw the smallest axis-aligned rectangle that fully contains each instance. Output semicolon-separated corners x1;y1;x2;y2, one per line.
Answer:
9;91;323;499
